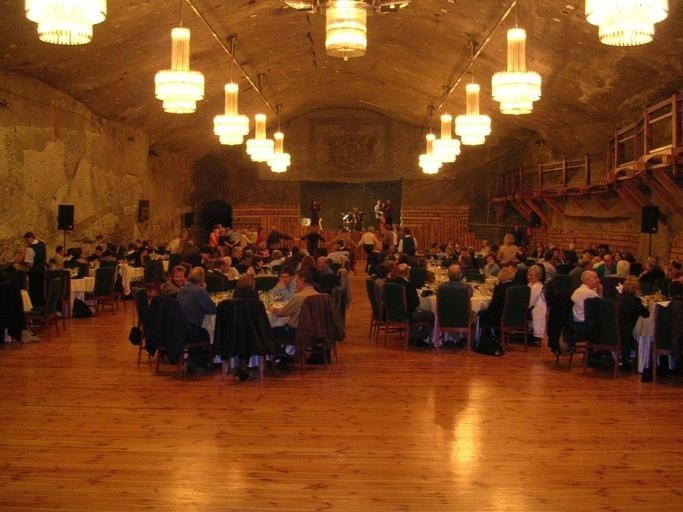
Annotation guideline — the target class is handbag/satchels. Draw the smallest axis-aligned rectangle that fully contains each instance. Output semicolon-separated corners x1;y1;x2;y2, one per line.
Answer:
128;288;142;345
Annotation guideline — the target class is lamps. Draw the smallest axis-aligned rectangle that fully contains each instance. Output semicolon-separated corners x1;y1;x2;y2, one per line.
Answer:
154;0;205;113
213;36;249;146
267;103;292;173
25;1;106;45
456;42;490;146
246;72;274;161
282;1;410;58
492;1;541;115
585;0;669;47
419;112;442;175
432;87;461;163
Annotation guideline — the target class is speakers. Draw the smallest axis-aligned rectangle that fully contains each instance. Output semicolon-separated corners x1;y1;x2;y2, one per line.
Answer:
531;213;540;225
184;213;193;226
57;205;75;230
641;205;659;233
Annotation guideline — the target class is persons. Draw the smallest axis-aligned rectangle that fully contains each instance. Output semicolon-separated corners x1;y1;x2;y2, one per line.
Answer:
533;243;578;281
658;281;683;376
99;251;118;269
483;255;499;277
269;267;300;300
164;267;188;293
52;245;72;271
374;198;392;231
510;224;525;245
176;267;216;377
583;249;600;264
613;277;654;382
0;280;39;344
656;260;683;293
526;265;546;339
296;226;325;254
207;225;265;252
165;235;184;267
316;255;338;287
611;260;630;277
265;225;294;251
183;240;200;265
299;257;317;274
308;201;323;227
639;257;659;282
186;247;303;278
327;226;358;271
355;226;382;272
396;230;418;257
384;255;426;271
595;255;616;278
232;273;259;380
423;238;497;267
270;271;320;354
570;270;613;368
120;237;155;266
372;264;394;322
440;265;472;344
386;264;435;347
622;254;642;274
523;226;534;247
513;253;527;283
569;261;591;286
495;234;523;265
475;267;516;346
597;243;610;258
18;232;45;308
63;248;88;276
87;245;104;261
381;225;397;250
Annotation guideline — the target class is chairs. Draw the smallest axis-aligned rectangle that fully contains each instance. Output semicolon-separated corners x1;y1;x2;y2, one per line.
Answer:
366;279;384;345
583;297;637;377
128;287;152;367
298;294;345;372
500;286;531;352
143;256;163;284
382;283;433;351
541;278;555;351
650;304;683;382
25;278;64;341
168;253;182;273
334;286;346;341
337;269;349;327
436;282;479;354
46;270;71;323
213;299;280;385
97;264;128;312
148;296;212;380
549;272;584;370
